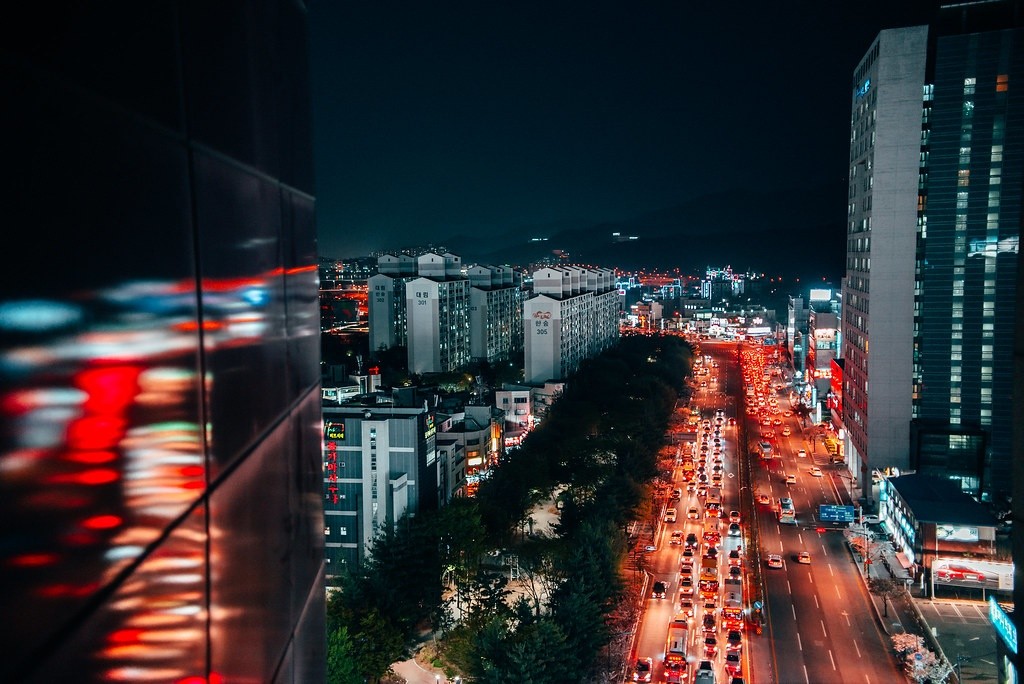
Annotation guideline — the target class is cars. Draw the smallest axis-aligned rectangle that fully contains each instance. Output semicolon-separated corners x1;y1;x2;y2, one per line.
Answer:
767;553;783;568
651;347;806;684
633;657;652;684
810;467;822;477
799;552;811;564
935;563;987;585
758;494;769;504
786;474;797;484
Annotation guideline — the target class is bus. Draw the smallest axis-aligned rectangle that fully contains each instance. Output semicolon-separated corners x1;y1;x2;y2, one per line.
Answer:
775;496;796;524
757;440;774;460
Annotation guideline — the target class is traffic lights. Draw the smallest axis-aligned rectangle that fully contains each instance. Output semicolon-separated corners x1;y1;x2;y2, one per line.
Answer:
817;528;825;532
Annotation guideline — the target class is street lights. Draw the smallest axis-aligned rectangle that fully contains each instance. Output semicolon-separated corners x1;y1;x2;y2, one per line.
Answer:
633;550;651;587
849;529;870;578
837;474;852;502
606;631;632;676
